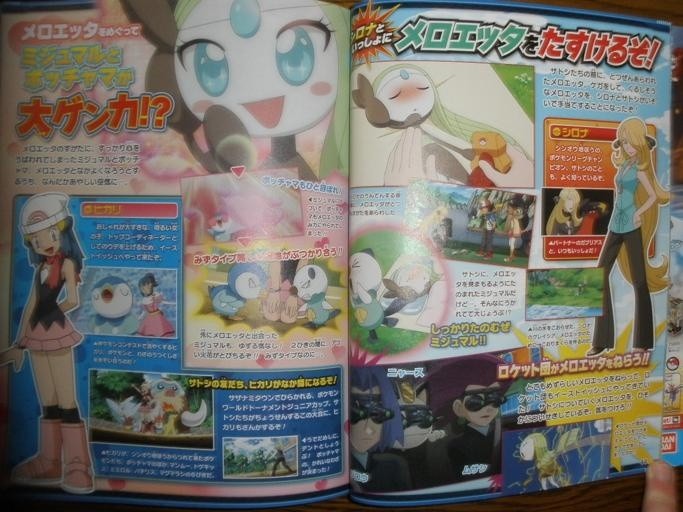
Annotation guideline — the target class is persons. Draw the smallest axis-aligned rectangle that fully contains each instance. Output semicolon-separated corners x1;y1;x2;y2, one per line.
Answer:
635;454;678;512
